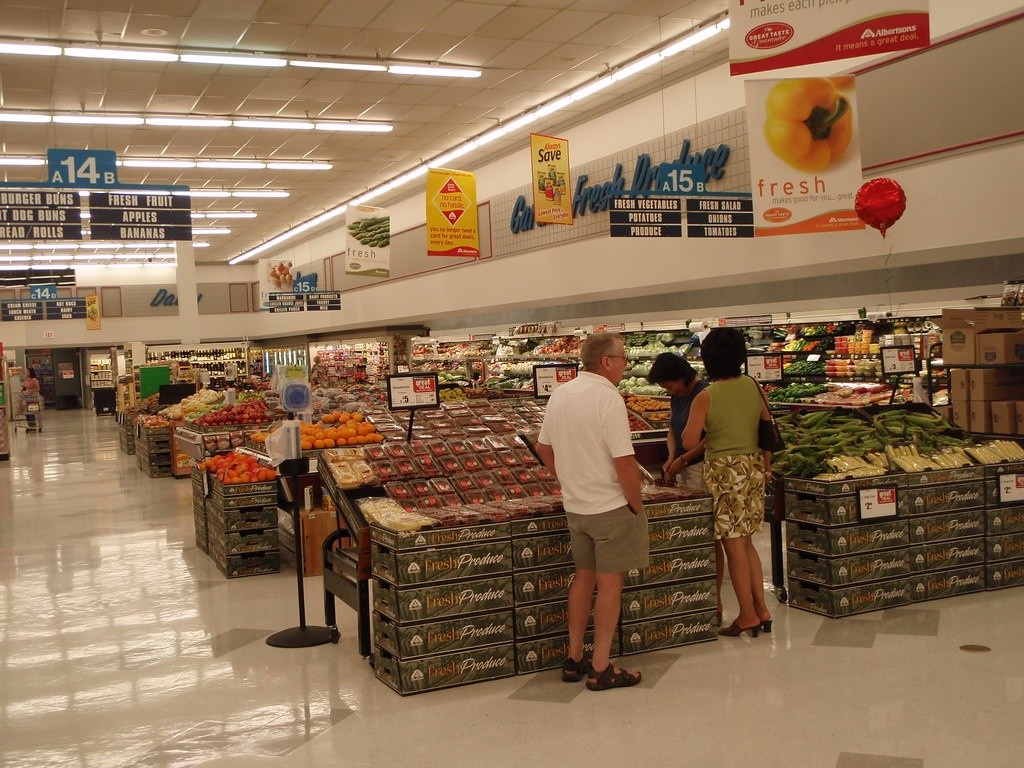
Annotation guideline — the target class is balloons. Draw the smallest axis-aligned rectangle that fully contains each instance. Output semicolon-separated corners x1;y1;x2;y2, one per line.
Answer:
853;178;907;240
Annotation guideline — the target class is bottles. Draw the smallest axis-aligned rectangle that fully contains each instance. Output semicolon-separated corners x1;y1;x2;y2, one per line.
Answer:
154;352;158;363
237;361;246;374
194;347;245;361
187;350;191;358
184;350;188;358
147;353;150;364
151;352;154;363
250;362;262;375
549;167;556;185
161;351;178;360
182;350;184;358
558;176;566;194
194;362;225;376
178;350;181;358
249;349;262;362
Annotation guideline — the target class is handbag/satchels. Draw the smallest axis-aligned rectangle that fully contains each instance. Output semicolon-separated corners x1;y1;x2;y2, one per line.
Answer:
746;374;785;452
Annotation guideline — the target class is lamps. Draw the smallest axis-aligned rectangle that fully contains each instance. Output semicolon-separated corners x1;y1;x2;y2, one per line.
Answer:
0;10;730;267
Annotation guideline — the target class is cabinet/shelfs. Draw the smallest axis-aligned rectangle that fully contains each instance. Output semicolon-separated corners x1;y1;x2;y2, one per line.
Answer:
0;356;10;461
85;342;308;418
309;315;953;427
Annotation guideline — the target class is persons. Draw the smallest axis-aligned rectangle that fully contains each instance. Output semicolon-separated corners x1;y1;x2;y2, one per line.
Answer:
647;327;772;637
21;368;40;431
537;332;649;691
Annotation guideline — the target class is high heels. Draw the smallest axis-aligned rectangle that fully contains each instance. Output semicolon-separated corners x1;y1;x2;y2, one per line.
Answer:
760;619;773;633
718;619;762;638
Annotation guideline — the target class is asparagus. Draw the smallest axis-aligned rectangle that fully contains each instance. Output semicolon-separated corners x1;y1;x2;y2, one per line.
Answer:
346;214;390;248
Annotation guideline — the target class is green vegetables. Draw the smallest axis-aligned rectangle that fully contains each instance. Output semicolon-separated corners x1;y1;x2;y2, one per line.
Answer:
767;382;826;403
783;360;826;375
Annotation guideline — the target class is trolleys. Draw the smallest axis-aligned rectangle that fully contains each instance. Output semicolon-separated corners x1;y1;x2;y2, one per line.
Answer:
12;392;44;434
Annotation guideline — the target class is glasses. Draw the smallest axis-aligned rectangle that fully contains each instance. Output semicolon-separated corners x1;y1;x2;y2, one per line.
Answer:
600;354;629;365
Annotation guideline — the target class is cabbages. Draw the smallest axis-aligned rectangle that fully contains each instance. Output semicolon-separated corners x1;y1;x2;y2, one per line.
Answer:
615;343;690;396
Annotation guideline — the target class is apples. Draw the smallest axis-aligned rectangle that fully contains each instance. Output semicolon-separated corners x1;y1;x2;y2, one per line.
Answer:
196;400;273;425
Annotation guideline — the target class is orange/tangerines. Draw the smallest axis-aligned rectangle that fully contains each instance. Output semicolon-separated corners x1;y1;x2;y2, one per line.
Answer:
250;411;385;451
198;451;276;485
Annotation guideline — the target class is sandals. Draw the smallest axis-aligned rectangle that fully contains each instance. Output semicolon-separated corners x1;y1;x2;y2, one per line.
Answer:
585;663;642;691
560;654;592;682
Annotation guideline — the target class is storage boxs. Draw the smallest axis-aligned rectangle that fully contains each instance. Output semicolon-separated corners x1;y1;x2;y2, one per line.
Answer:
117;412;136;455
784;460;1024;619
928;306;1024;435
193;396;721;697
133;421;173;478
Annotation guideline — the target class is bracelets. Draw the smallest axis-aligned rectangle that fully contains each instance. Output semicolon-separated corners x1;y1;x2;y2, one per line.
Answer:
680;455;688;465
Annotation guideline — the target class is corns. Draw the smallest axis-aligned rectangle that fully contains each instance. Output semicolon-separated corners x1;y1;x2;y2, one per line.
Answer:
771;408;1024;482
